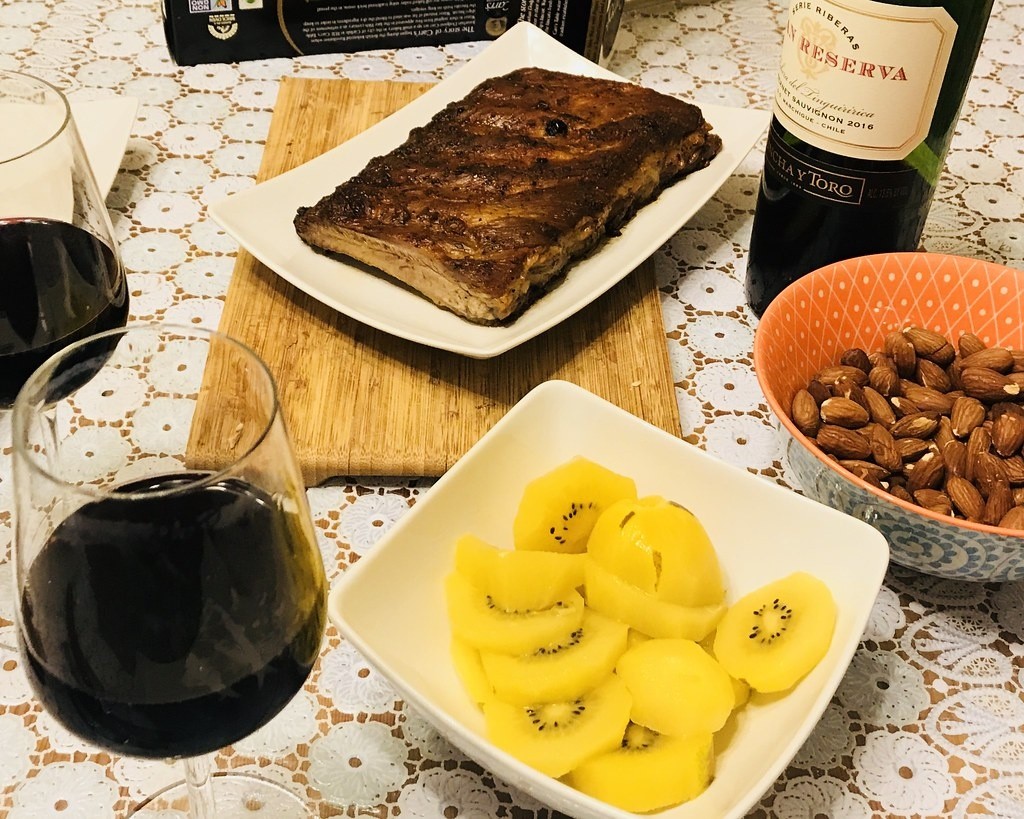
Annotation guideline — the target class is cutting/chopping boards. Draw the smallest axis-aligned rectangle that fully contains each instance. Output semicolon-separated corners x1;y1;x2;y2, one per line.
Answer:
187;75;684;484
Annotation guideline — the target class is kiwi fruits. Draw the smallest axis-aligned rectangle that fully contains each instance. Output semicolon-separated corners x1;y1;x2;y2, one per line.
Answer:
446;456;838;810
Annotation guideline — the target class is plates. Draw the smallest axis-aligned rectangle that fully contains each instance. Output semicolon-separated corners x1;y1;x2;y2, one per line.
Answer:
0;96;132;215
205;19;774;359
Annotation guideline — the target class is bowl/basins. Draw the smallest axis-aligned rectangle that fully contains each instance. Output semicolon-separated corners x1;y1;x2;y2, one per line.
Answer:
753;252;1024;582
326;379;891;819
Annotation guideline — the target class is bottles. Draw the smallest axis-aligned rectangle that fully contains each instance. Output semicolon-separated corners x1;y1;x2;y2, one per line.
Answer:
743;0;994;320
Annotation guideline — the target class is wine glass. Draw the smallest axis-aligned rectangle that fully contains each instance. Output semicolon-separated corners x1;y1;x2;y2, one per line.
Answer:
0;68;132;467
12;322;329;819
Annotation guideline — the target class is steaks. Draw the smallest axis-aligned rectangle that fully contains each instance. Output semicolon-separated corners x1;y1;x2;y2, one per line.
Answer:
293;66;724;325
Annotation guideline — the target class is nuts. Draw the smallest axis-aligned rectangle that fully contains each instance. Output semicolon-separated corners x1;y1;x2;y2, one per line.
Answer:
793;327;1024;533
